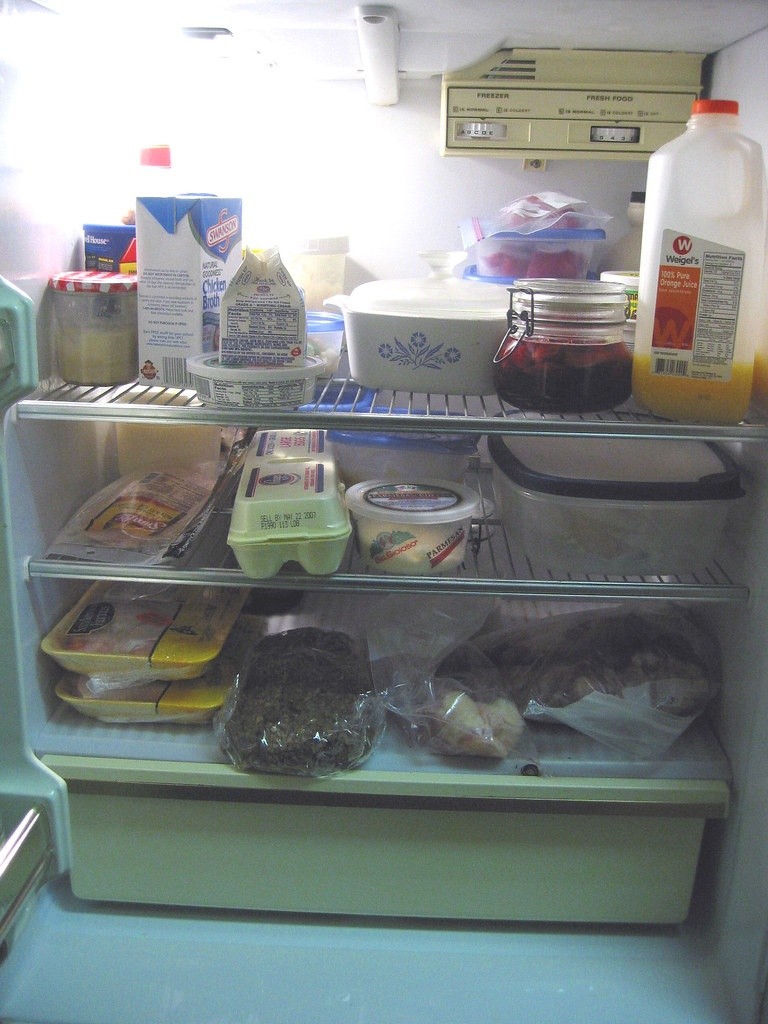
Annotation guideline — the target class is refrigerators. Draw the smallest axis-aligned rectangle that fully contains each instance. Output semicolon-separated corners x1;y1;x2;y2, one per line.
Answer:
0;0;768;1024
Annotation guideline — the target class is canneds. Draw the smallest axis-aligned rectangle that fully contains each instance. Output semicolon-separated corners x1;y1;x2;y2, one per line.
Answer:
601;271;640;323
51;272;138;385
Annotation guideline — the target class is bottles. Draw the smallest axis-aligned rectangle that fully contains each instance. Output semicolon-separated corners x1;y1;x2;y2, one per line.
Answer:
123;145;187;224
632;98;767;424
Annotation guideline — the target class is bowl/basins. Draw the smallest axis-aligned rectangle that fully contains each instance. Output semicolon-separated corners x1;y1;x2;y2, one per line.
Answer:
306;312;344;374
345;476;482;574
